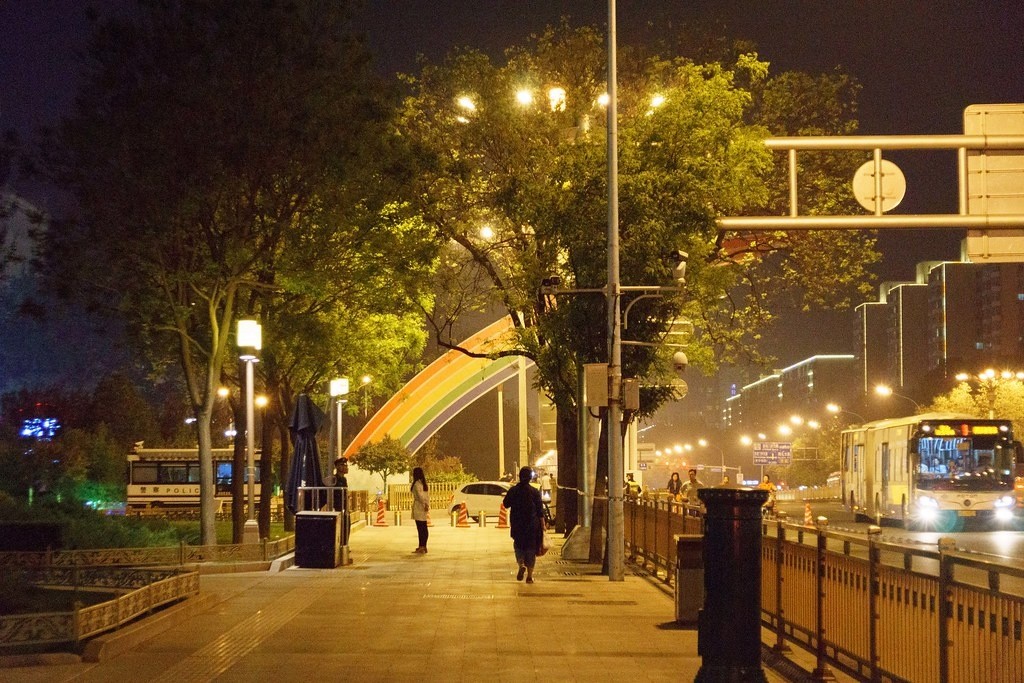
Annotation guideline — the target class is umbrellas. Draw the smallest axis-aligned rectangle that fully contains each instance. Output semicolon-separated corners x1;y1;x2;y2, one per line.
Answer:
285;393;327;515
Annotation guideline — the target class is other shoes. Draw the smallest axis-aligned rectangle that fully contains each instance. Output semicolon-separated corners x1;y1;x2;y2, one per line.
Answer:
517;567;526;581
412;547;427;553
526;578;534;584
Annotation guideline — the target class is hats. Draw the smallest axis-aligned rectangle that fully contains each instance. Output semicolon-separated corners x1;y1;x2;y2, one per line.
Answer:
626;471;633;477
334;458;347;464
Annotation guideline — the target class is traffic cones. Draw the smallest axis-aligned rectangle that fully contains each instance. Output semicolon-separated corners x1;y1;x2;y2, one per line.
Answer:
495;503;510;528
803;503;815;527
456;502;471;527
427;507;434;527
373;498;389;527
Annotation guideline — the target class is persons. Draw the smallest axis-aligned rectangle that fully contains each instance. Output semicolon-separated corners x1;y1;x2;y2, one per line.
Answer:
667;472;683;514
724;476;729;484
410;467;430;554
930;455;963;473
333;457;350;545
534;472;557;497
680;469;704;517
758;475;778;492
503;466;547;583
625;473;641;495
500;472;512;482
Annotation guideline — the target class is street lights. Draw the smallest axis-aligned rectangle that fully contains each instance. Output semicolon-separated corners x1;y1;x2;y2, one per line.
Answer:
700;440;724;482
330;379;349;459
235;320;263;543
363;377;370;419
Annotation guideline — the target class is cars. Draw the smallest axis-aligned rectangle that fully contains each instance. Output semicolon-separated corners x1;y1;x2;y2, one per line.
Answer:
448;481;517;523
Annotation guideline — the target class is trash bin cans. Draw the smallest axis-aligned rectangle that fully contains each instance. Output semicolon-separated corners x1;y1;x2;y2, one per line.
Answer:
674;534;705;624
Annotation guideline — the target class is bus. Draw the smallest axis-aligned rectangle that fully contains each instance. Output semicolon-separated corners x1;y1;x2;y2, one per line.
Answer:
841;414;1024;531
127;441;306;520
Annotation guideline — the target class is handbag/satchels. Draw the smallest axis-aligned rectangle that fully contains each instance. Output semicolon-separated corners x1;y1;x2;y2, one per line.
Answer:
535;530;549;557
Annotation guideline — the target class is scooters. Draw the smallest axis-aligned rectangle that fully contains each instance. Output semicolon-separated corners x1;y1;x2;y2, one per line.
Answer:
762;491;777;520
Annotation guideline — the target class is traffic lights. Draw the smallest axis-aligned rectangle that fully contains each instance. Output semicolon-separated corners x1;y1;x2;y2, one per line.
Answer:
680;458;689;471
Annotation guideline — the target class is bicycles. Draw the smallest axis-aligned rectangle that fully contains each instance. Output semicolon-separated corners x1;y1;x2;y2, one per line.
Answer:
542;500;556;530
